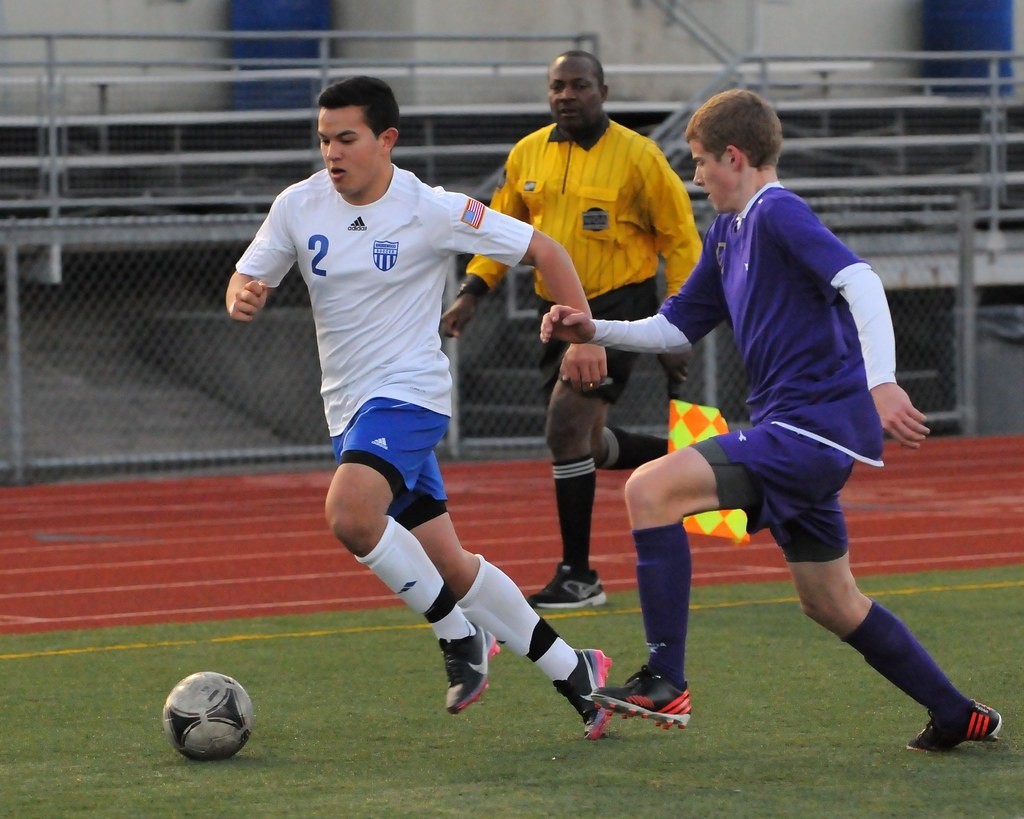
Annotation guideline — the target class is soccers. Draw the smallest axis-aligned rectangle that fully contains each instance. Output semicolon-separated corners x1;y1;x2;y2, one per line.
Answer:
162;671;254;762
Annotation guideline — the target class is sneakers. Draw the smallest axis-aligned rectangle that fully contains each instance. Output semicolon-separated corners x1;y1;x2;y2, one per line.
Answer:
438;621;501;715
552;648;614;741
905;699;1002;751
590;664;692;730
527;560;607;609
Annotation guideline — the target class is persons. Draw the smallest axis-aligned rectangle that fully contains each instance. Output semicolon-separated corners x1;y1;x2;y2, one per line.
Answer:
438;49;704;609
225;75;613;742
540;88;1002;753
